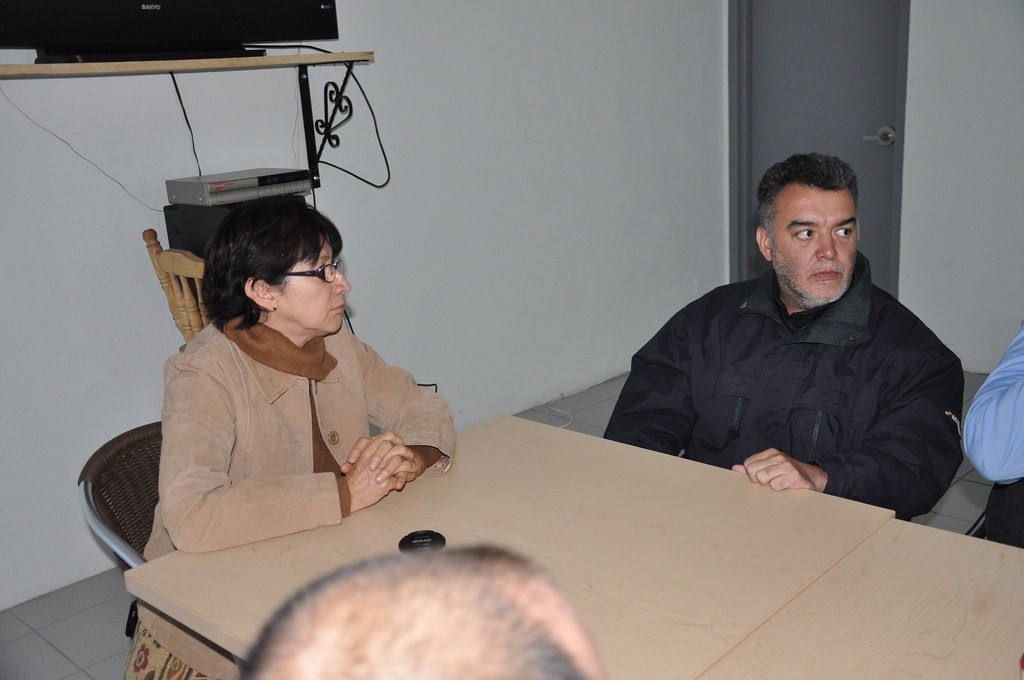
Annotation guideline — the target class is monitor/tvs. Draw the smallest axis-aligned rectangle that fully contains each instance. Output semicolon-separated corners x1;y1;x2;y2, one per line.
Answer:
0;0;340;64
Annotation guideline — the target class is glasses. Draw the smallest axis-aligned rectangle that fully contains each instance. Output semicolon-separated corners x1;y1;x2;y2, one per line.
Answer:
283;259;344;283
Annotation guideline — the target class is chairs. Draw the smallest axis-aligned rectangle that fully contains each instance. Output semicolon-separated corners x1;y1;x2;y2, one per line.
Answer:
78;420;161;572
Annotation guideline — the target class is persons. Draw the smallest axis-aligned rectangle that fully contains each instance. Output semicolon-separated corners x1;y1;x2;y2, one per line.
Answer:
601;153;964;522
240;544;609;680
142;198;456;562
964;317;1024;548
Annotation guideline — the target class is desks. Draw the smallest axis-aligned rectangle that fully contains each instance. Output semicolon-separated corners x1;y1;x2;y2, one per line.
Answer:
124;416;895;680
696;519;1024;680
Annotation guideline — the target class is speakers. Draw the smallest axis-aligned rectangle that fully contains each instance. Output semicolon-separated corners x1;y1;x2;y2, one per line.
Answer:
162;194;305;308
